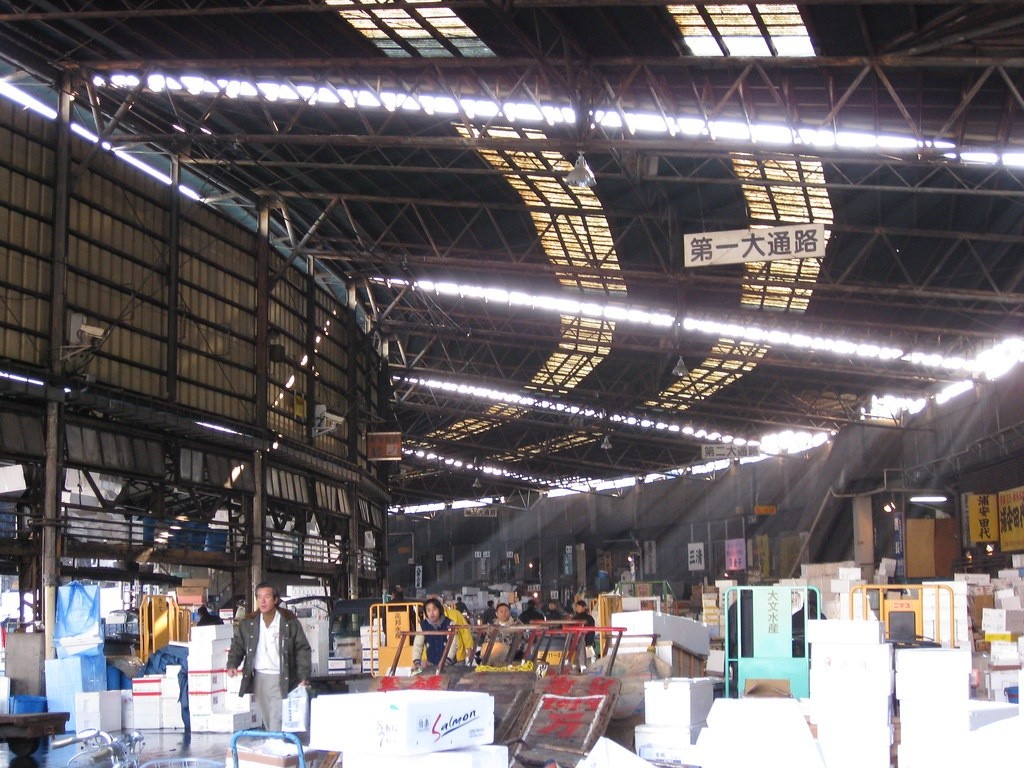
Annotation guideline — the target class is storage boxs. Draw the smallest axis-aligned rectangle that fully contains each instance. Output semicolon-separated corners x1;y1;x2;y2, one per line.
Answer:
0;465;143;545
0;555;1024;768
265;515;376;571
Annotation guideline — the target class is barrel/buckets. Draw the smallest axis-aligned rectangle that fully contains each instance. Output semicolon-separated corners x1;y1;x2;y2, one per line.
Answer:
8;694;46;715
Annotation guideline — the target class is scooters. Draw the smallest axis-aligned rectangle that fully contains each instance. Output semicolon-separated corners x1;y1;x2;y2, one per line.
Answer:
49;727;225;768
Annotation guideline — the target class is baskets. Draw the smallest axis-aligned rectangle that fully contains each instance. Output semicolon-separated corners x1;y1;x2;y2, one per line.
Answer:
139;758;225;768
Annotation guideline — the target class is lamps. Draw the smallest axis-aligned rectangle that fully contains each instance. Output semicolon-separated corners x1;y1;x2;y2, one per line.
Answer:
672;355;689;376
473;477;482;487
566;150;597;187
601;437;612;449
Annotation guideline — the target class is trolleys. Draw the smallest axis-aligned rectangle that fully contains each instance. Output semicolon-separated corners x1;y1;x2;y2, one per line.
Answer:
229;729;311;768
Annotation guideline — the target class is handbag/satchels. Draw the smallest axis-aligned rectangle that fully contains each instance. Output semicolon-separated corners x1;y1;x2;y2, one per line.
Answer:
282;684;310;732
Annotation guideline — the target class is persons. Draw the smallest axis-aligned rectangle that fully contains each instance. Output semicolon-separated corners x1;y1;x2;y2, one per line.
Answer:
382;589;416;647
412;593;594;673
197;607;224;626
226;582;311;741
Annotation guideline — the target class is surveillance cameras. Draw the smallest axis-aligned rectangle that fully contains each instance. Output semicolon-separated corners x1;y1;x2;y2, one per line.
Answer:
80;325;111;339
324;412;344;424
